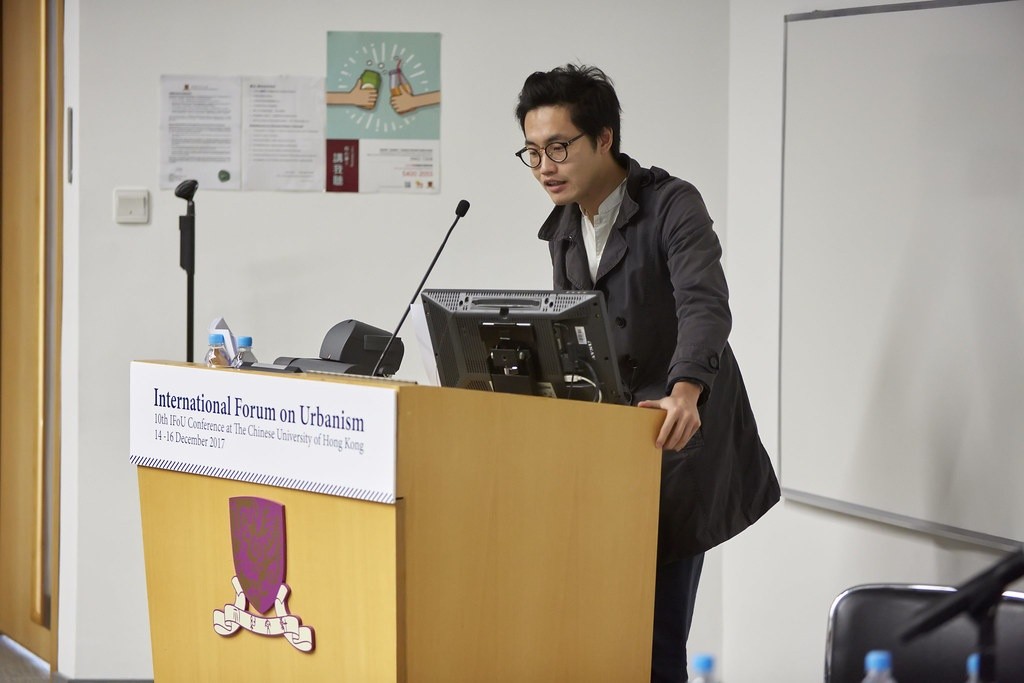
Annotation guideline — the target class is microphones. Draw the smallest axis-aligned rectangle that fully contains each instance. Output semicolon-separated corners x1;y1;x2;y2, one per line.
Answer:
371;200;470;377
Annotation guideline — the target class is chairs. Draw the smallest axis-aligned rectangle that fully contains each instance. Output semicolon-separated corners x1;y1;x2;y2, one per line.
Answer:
824;583;1024;683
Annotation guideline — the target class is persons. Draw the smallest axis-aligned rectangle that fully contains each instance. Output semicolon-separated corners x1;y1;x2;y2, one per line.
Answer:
513;63;782;683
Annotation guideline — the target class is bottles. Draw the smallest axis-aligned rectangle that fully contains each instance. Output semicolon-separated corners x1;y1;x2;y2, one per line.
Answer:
231;336;259;369
966;654;983;683
205;333;232;369
860;650;896;682
688;654;722;683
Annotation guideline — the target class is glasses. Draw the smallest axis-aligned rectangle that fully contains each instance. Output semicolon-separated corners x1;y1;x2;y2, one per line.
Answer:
514;129;595;169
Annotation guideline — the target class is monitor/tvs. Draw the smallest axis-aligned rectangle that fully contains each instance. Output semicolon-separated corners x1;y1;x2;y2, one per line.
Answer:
421;288;630;407
